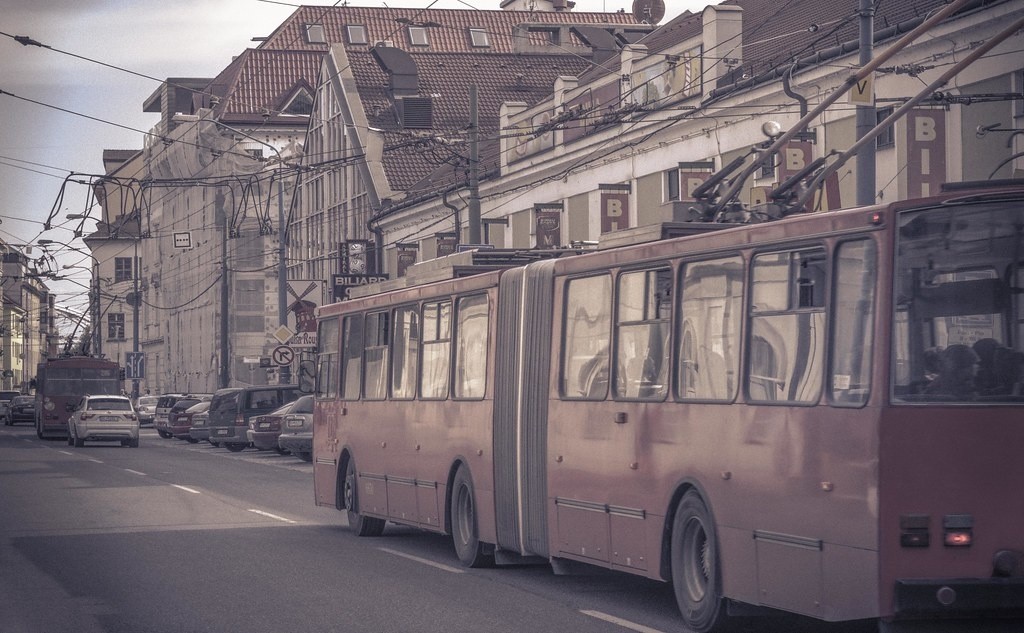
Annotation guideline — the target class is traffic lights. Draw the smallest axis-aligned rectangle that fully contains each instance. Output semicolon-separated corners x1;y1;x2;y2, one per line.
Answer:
120;369;125;380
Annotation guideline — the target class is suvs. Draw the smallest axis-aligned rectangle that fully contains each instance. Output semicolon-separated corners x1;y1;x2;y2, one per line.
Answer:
65;394;140;448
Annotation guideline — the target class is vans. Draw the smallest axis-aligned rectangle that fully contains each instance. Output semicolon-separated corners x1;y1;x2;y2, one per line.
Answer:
208;385;315;452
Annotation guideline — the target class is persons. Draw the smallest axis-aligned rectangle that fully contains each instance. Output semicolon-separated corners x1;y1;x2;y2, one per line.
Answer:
922;338;1012;402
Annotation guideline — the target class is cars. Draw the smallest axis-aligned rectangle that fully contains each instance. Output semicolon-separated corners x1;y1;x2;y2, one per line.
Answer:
134;398;161;427
168;395;213;440
278;395;314;462
171;403;211;443
153;394;214;439
190;410;226;448
3;396;35;426
0;390;21;420
246;402;295;456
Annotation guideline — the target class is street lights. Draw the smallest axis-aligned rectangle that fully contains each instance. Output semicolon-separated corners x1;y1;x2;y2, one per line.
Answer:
51;277;91;319
38;240;101;358
66;214;139;398
62;265;97;354
172;114;292;385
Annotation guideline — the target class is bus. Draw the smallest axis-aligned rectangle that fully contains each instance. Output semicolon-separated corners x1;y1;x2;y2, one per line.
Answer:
35;353;121;439
312;189;1024;632
